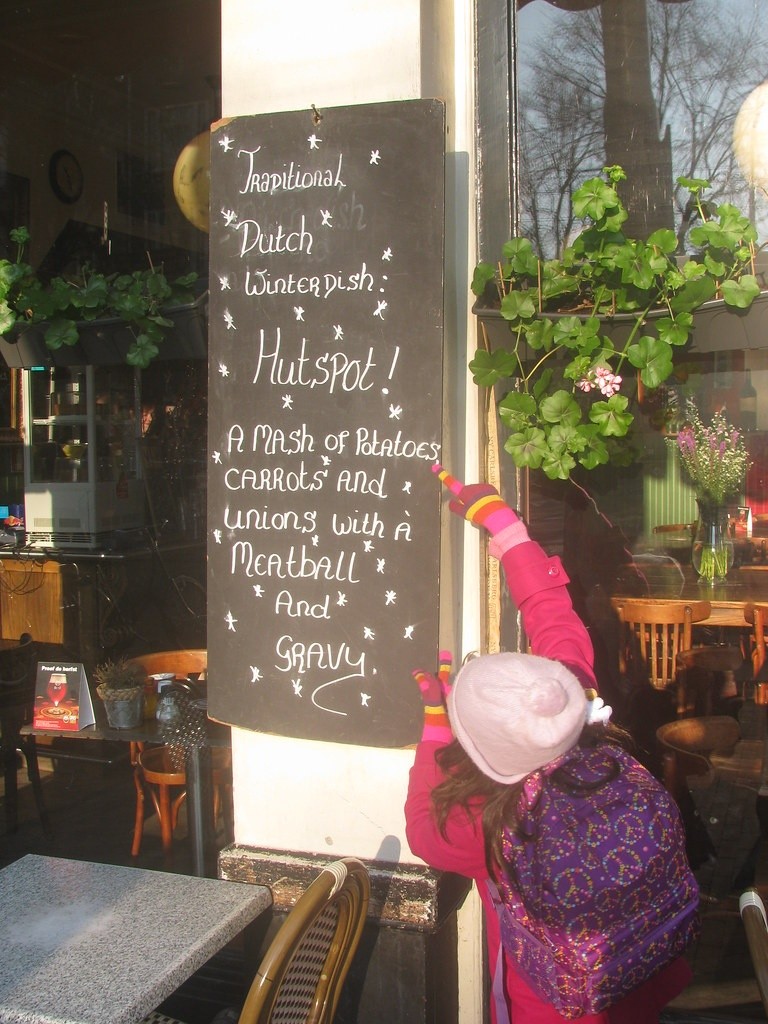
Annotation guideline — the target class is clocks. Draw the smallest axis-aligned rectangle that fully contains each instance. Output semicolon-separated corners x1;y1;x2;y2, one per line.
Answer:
48;148;85;204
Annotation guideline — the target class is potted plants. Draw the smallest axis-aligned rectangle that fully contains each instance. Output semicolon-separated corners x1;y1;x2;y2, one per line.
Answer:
94;655;149;729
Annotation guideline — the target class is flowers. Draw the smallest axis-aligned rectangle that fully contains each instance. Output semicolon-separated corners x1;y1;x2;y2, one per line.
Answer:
678;413;756;579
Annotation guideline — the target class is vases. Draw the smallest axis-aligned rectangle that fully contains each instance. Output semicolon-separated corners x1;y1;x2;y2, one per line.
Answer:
690;498;738;581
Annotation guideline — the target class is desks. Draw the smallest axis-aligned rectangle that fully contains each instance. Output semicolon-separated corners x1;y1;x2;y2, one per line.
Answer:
0;843;273;1024
611;564;768;700
20;701;232;837
654;530;768;549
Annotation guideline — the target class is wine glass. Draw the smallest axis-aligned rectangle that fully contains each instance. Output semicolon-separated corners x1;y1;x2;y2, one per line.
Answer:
58;440;89;482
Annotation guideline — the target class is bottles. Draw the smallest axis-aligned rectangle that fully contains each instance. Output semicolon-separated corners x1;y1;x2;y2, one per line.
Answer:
720;399;729;427
143;677;158;719
740;368;758;431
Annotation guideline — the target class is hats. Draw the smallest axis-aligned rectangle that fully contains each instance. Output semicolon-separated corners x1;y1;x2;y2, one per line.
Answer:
447;653;586;786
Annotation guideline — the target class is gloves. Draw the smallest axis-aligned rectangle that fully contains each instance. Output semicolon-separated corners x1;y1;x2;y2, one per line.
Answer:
431;464;532;560
412;652;457;745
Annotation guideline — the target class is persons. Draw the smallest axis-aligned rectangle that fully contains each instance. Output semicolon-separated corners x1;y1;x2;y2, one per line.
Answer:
405;465;695;1024
524;475;648;688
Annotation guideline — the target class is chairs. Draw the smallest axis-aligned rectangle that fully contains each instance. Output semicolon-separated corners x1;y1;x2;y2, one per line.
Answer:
614;602;767;923
126;648;233;856
144;856;375;1024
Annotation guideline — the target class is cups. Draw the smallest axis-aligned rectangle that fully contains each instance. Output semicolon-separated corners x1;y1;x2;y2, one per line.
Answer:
150;672;175;693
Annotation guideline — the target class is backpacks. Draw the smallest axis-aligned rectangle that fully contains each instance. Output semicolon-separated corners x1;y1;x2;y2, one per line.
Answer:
489;745;702;1020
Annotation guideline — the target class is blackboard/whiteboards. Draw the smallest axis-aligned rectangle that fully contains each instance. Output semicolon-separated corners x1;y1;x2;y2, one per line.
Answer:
203;97;445;748
